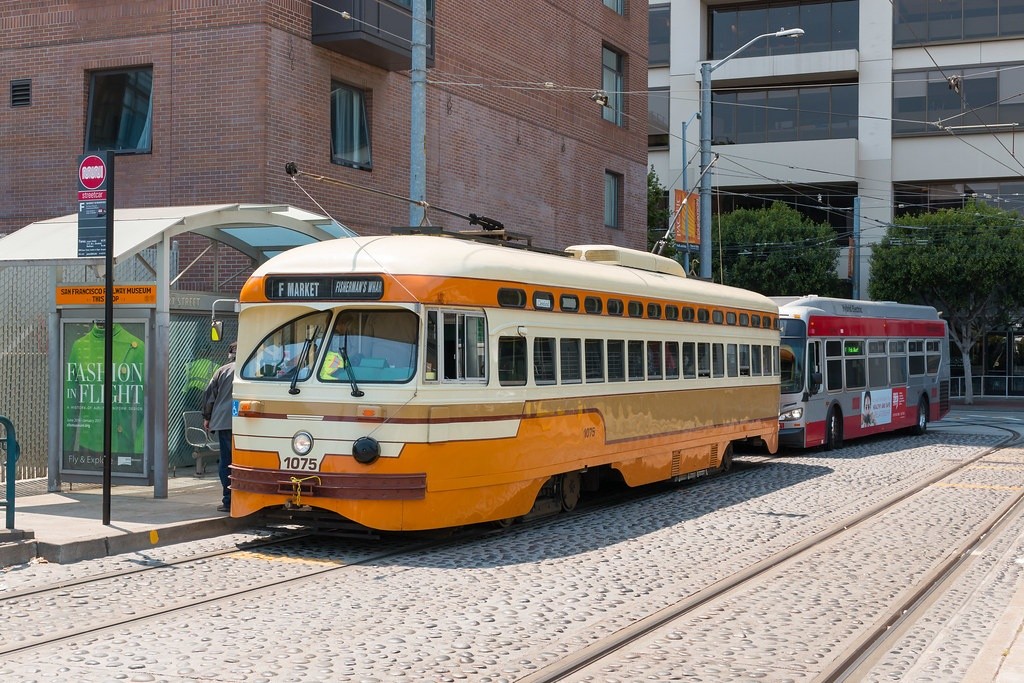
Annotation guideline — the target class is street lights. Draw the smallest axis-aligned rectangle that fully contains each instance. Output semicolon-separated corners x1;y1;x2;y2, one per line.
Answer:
698;26;806;278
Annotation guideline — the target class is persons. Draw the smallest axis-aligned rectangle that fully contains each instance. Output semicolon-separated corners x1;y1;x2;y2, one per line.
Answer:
861;391;878;428
201;341;236;512
259;309;438;384
177;337;224;453
62;321;146;456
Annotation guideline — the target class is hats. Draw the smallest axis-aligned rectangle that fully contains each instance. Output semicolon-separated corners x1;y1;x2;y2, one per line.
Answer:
228;342;237;353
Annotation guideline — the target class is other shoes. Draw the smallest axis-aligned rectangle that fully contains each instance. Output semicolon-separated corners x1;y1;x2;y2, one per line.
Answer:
217;505;230;512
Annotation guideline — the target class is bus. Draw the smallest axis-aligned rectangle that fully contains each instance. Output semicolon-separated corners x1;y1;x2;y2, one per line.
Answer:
227;160;781;541
753;293;949;451
